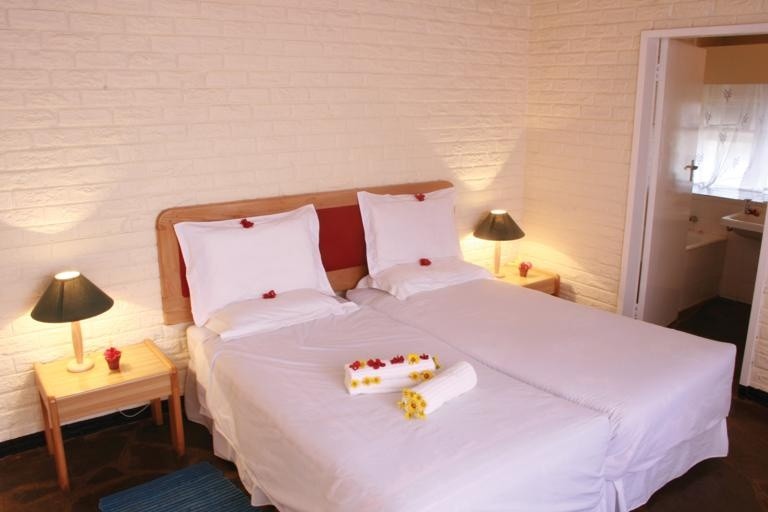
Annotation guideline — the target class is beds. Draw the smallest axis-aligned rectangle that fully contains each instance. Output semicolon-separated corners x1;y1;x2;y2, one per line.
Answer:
155;179;736;512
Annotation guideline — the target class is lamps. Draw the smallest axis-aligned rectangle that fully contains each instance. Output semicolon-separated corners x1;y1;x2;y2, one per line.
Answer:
473;209;525;279
31;268;114;375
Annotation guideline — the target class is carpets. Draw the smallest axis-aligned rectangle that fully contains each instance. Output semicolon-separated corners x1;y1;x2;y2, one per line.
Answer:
94;457;263;511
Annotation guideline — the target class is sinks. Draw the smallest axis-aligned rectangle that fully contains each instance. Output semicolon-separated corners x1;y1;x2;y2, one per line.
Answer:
721;211;764;240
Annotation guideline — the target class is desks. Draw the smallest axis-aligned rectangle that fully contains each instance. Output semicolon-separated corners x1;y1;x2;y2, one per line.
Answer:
33;339;185;492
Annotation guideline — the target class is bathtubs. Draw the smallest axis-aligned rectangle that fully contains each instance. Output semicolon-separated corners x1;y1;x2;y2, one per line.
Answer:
686;230;728;252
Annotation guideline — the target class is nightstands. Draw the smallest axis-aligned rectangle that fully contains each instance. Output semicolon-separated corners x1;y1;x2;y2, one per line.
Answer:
493;264;560;298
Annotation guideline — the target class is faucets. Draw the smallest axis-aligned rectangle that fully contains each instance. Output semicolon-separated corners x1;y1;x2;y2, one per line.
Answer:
689;215;697;224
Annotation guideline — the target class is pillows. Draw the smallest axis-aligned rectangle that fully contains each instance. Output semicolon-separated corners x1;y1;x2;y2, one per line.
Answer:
173;203;335;328
355;187;463;272
356;256;492;300
205;287;361;343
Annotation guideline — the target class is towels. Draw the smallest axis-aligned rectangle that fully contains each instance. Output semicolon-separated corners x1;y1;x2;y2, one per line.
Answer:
344;355;479;422
